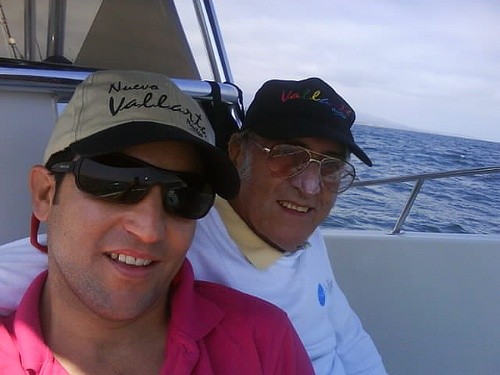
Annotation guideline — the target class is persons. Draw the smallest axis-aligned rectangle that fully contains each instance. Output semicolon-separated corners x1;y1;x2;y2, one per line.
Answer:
2;67;316;375
1;76;388;374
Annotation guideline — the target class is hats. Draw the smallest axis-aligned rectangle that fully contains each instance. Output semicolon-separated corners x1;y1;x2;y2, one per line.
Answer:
44;68;241;201
240;77;373;167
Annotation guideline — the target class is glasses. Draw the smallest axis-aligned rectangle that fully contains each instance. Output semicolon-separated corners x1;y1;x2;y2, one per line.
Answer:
247;136;358;194
45;148;216;221
